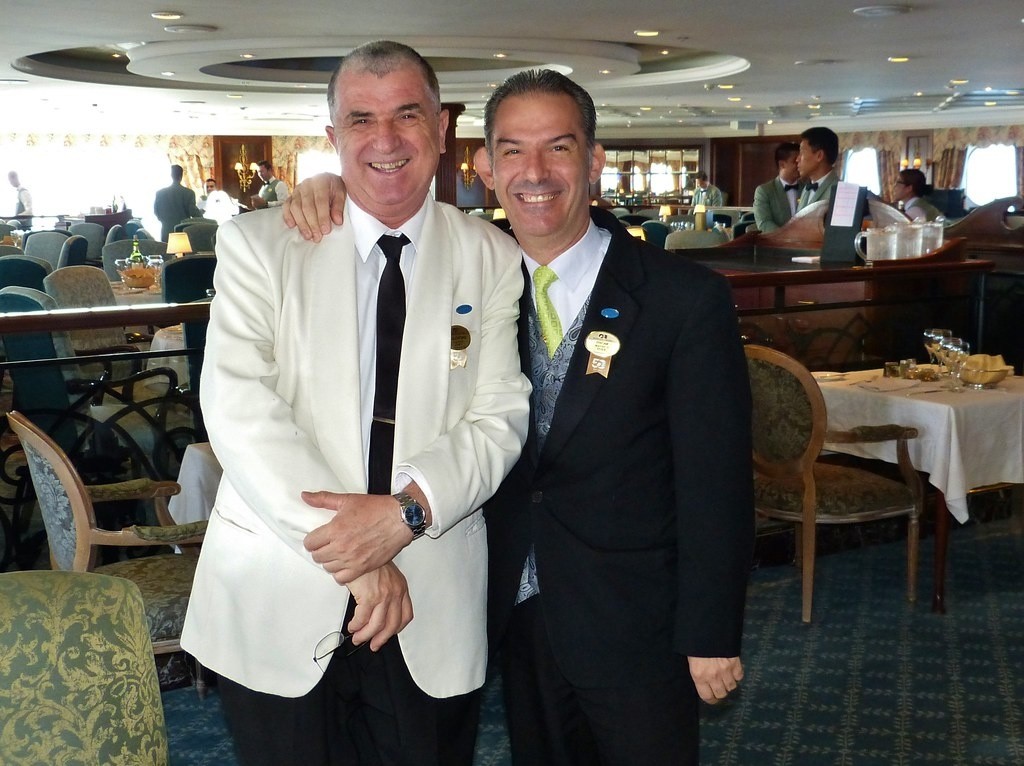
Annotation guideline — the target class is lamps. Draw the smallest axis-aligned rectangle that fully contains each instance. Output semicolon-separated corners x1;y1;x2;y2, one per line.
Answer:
234;144;258;194
166;231;193;259
659;205;671;223
461;146;477;189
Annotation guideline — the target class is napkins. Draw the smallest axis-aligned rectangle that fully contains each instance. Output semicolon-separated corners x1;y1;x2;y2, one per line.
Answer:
855;377;920;392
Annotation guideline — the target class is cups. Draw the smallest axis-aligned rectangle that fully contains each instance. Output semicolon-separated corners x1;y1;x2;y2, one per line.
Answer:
10;229;24;248
90;206;103;214
115;255;163;296
854;224;898;266
922;216;946;254
897;217;924;259
669;221;726;234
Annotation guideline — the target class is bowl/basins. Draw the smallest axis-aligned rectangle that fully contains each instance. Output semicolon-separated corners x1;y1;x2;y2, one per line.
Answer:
959;368;1008;388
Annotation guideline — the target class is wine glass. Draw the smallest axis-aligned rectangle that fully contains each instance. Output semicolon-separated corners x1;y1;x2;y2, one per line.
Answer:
924;329;970;392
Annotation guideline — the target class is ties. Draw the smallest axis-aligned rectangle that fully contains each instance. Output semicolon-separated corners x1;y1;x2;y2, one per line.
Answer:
533;265;565;358
366;230;407;496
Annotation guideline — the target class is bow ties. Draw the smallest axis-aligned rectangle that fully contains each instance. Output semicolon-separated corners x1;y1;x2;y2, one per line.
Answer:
784;183;800;191
806;181;820;193
701;190;707;193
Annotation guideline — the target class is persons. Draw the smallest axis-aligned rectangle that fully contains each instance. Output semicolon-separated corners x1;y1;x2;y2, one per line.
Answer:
3;171;33;232
894;169;948;227
197;178;216;216
180;41;534;766
153;165;203;243
687;172;722;215
281;69;757;766
251;159;288;210
752;143;812;233
797;127;841;212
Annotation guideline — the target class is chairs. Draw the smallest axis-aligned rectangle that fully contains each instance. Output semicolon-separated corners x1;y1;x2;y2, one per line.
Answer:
0;209;219;766
609;208;758;252
742;345;924;623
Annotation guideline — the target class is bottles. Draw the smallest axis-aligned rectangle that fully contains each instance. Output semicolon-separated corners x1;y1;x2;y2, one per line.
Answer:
105;195;127;215
128;235;143;263
884;359;942;381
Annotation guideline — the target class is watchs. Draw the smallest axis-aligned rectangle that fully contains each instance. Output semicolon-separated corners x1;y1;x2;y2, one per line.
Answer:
394;492;427;540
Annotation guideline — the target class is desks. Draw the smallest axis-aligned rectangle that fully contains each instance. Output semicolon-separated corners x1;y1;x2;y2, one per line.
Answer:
147;325;194;431
165;443;223;555
108;281;162;345
817;363;1024;614
